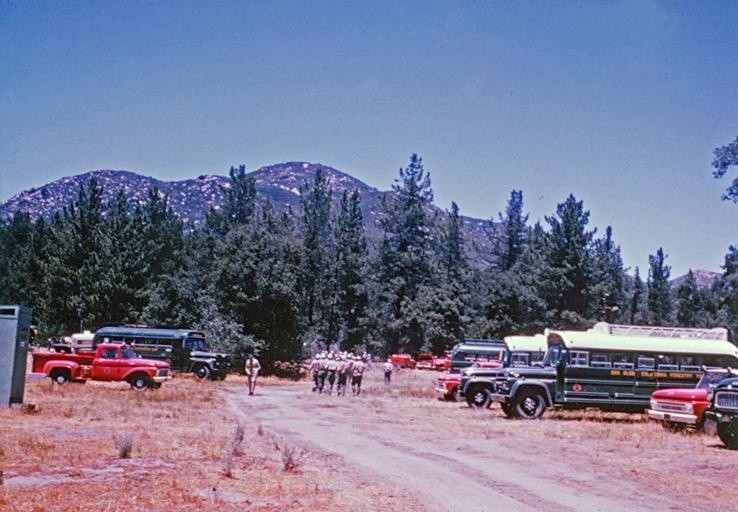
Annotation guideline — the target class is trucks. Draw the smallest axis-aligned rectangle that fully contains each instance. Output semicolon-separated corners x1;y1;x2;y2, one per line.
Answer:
26;322;234;395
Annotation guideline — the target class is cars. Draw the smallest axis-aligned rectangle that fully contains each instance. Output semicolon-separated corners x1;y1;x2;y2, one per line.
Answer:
433;359;502;403
645;368;738;436
391;349;453;372
703;377;738;451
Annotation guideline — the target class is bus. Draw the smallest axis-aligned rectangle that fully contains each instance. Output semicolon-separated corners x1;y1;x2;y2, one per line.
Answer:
455;322;737;421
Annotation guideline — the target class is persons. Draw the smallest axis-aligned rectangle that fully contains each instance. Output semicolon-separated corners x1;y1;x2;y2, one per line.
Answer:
310;348;372;396
383;358;393;385
245;351;262;396
